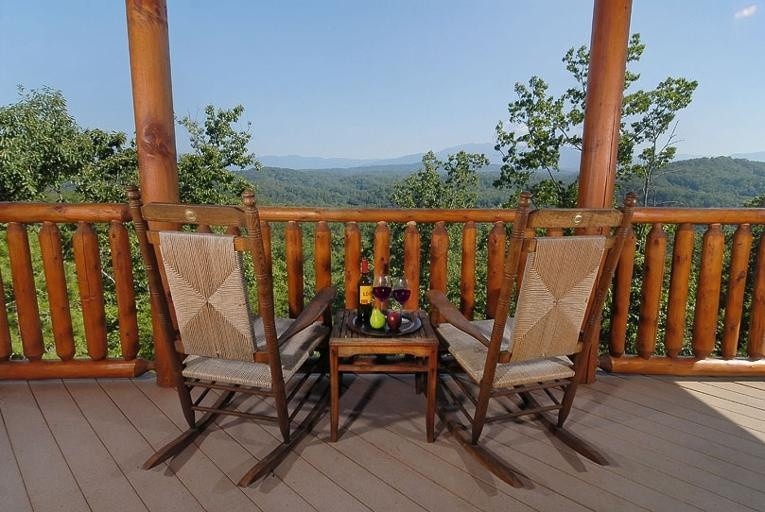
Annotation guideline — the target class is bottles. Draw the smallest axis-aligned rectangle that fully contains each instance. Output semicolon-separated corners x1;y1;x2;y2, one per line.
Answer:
357;260;373;326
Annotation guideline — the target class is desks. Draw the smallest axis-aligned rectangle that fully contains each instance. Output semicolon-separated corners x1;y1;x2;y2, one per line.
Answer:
327;307;439;442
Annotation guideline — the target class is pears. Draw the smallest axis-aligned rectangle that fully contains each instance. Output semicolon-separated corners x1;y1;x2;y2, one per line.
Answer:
369;307;386;330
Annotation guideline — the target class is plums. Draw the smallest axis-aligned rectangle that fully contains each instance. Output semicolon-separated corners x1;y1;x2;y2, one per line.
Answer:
388;312;401;328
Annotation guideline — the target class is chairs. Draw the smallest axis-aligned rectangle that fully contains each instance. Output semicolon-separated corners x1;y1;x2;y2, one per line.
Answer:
125;183;339;488
425;192;638;488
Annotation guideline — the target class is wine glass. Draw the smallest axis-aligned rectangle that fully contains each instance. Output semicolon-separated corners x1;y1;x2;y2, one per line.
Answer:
373;273;411;324
